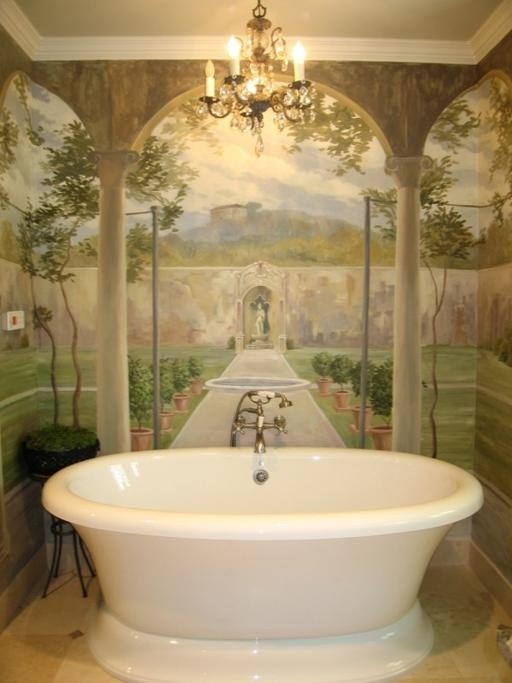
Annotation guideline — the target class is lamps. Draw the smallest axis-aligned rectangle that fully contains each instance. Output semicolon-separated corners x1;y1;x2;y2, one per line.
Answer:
197;1;317;156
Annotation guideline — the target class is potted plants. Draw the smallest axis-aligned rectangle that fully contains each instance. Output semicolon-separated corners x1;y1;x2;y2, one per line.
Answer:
18;426;99;475
311;351;394;450
128;353;204;452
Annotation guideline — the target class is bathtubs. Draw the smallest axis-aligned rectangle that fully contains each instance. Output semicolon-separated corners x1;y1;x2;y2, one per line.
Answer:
40;446;484;683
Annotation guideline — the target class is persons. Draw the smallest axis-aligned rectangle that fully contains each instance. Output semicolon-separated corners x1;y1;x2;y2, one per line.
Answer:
254;303;266;335
